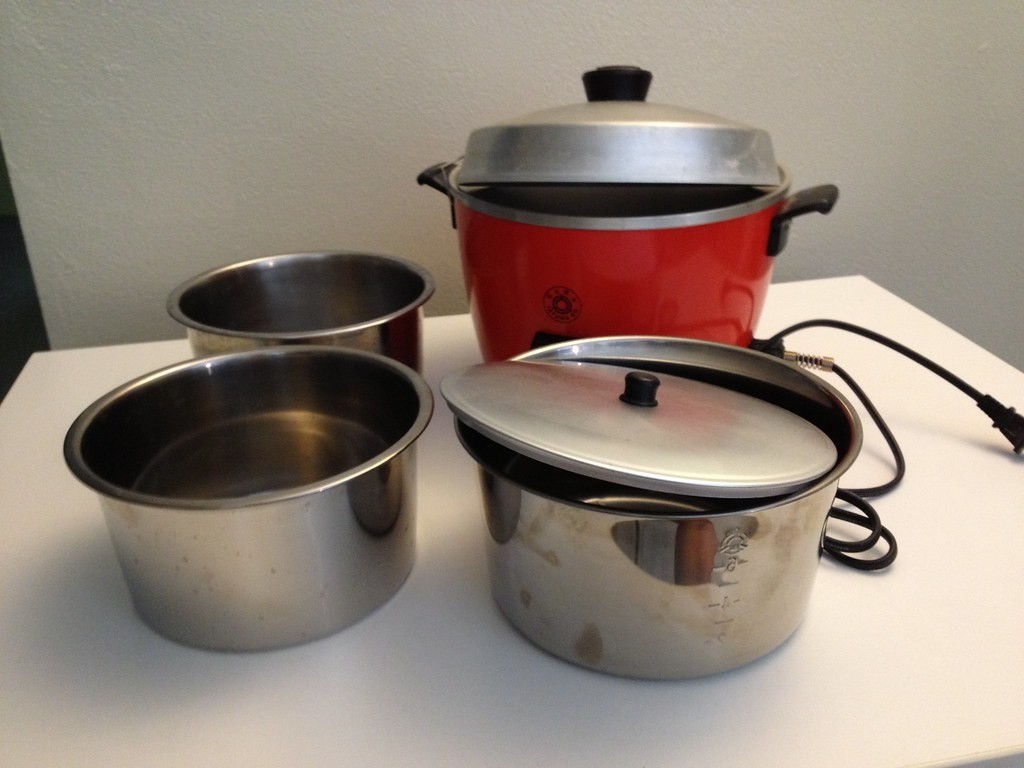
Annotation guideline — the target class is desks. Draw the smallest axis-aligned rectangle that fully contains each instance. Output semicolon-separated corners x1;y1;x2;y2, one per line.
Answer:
0;275;1024;768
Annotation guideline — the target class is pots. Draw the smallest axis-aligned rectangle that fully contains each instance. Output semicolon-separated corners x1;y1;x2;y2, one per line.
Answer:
165;252;434;374
66;349;434;653
418;67;839;361
458;336;864;683
